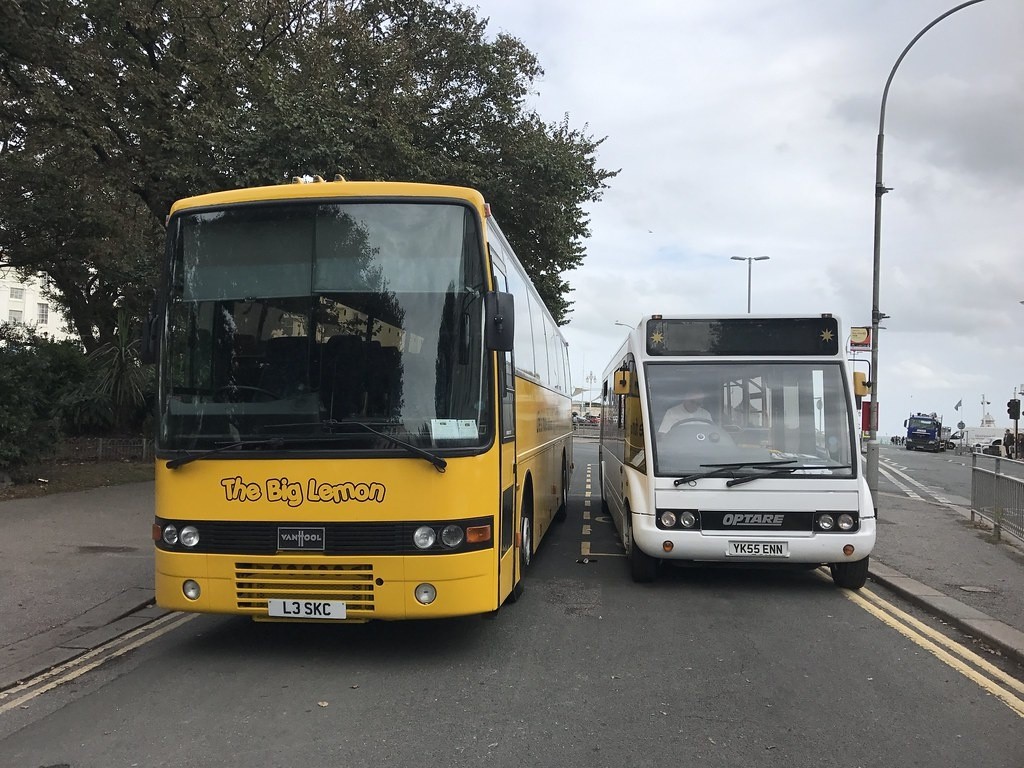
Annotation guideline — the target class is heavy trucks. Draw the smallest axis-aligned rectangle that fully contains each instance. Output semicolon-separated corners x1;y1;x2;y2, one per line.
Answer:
904;412;951;453
947;427;1008;451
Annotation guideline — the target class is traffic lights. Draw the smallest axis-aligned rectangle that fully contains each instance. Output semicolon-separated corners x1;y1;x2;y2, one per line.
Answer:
1007;399;1020;420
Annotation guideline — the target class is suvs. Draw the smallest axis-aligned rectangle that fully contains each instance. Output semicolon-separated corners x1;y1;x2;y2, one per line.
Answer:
971;436;1005;455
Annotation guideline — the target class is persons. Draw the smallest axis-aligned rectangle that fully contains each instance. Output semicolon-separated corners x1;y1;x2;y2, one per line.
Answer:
657;389;714;439
1003;429;1024;461
891;436;906;447
573;415;577;430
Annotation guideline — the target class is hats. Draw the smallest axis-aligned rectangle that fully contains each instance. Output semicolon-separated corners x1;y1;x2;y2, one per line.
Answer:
1005;429;1010;432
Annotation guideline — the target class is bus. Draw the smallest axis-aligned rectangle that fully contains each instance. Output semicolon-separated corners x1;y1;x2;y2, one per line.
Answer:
598;312;877;589
149;175;576;625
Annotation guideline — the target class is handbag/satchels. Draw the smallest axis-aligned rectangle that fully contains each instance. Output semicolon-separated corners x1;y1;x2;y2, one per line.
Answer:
1009;446;1015;455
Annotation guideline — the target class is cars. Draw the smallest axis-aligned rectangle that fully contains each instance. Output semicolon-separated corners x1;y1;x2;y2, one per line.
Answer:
572;414;600;427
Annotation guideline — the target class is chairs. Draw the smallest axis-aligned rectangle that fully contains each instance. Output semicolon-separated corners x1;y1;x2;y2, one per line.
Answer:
382;345;423;417
327;335;368;422
653;389;687;432
364;340;389;418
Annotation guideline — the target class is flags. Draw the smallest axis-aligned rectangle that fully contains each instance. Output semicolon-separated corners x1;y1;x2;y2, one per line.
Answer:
954;401;963;412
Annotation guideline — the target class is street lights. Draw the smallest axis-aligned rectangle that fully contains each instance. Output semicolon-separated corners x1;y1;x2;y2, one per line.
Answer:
731;255;770;313
